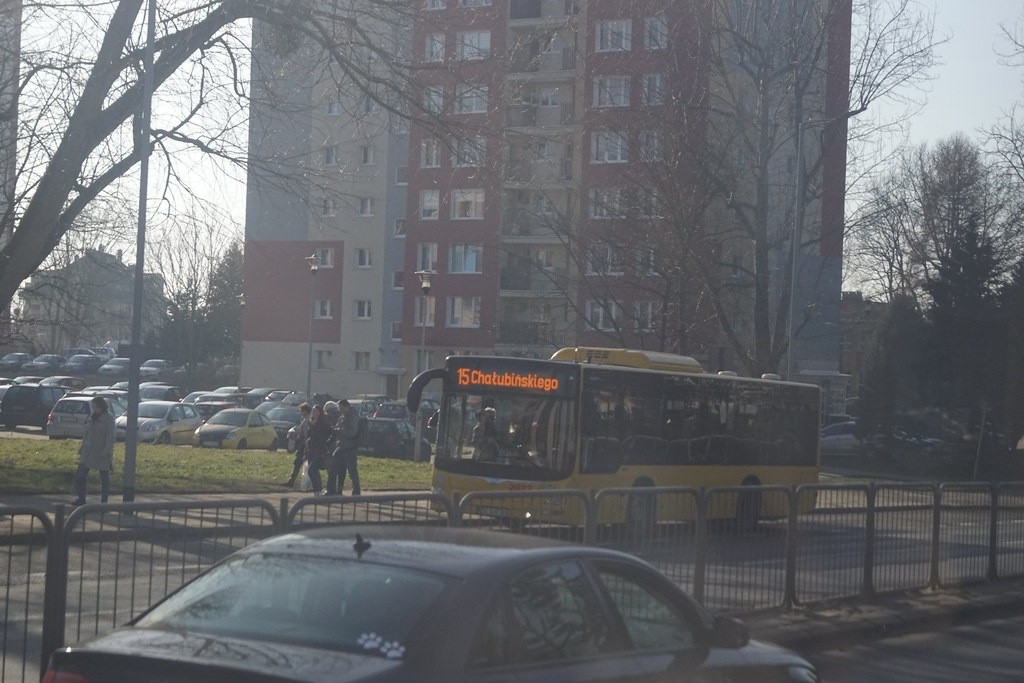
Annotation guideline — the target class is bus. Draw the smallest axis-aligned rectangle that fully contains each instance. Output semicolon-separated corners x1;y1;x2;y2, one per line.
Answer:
405;342;822;538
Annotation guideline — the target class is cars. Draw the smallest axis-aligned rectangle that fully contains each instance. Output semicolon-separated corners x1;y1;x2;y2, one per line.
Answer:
38;522;818;682
1;338;441;463
821;421;876;455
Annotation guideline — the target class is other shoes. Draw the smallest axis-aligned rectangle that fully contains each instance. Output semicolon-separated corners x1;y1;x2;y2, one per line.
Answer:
280;481;361;504
71;498;86;506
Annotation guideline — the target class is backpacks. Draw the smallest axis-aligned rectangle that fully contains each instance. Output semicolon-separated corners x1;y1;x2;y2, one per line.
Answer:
350;412;368;446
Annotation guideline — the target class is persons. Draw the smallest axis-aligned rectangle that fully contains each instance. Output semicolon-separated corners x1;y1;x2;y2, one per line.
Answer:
322;398;361;498
71;396;115;507
472;406;505;461
303;404;328;493
322;400;344;497
280;403;312;487
